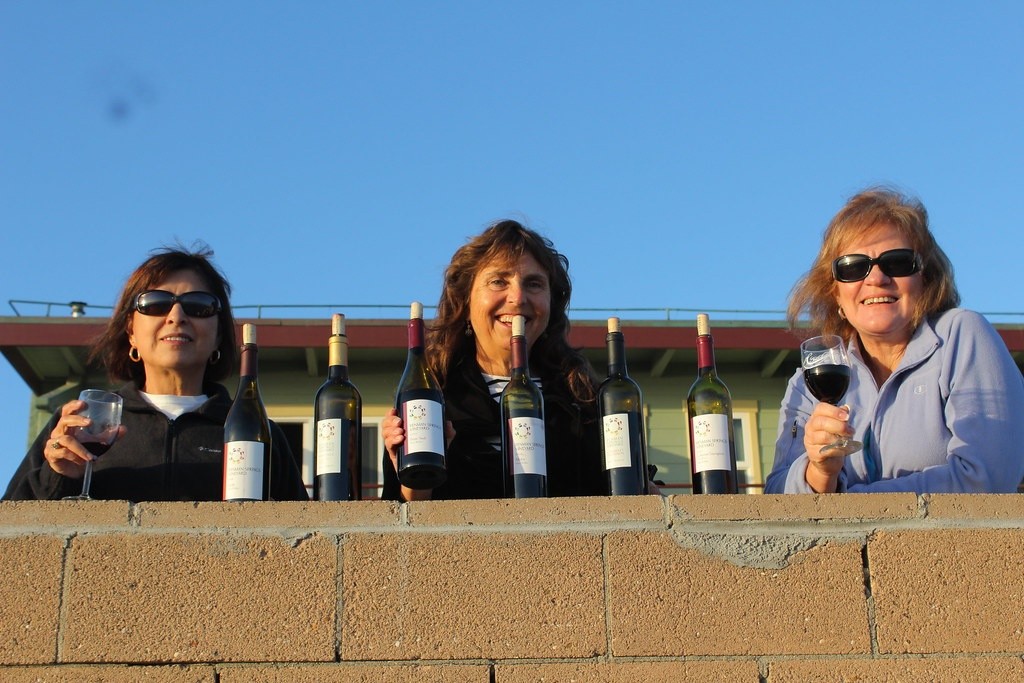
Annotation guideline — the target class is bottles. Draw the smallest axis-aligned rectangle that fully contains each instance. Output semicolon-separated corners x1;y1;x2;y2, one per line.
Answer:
500;316;548;498
222;323;272;500
687;315;739;494
314;314;362;501
395;302;449;491
596;318;649;495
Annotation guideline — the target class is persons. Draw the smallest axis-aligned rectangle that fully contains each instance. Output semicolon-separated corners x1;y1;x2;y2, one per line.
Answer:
762;184;1024;494
379;220;661;505
0;236;309;502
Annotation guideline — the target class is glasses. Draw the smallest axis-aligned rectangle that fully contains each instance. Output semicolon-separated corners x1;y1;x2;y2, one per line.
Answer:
128;289;223;320
829;249;920;283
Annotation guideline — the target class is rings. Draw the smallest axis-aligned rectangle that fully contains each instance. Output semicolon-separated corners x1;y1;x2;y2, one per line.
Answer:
51;436;62;449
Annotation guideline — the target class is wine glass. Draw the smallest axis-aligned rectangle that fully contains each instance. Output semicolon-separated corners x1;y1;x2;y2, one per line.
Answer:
800;334;864;455
61;389;123;500
